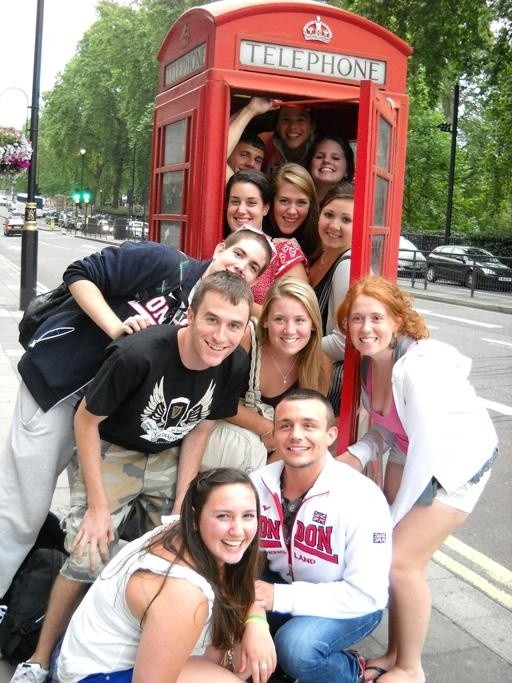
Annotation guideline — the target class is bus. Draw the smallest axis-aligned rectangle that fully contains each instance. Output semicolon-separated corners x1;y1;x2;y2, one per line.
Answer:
15;192;44;217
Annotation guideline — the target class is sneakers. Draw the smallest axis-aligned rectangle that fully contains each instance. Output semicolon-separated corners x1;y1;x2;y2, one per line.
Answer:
9;660;49;683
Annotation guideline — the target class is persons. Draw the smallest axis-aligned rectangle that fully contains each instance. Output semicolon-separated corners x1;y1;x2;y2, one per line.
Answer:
54;466;281;682
228;90;368;353
235;387;393;680
14;270;255;681
0;226;275;597
241;275;332;444
333;275;498;682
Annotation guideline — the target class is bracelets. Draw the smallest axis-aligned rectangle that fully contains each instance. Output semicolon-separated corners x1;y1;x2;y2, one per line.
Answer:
244;607;268;627
257;425;276;440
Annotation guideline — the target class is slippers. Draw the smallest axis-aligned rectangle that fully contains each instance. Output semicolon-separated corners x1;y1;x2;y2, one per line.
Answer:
362;658;386;682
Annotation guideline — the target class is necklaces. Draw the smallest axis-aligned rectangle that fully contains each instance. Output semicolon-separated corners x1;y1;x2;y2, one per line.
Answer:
270;348;298;383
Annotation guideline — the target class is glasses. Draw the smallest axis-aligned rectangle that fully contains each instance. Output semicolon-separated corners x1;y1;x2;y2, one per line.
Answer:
236;223;278;264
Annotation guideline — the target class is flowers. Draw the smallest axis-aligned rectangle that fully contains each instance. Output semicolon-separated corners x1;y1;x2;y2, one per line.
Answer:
0;126;33;178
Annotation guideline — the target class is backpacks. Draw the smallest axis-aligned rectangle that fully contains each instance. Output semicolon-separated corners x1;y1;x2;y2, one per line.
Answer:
0;548;75;663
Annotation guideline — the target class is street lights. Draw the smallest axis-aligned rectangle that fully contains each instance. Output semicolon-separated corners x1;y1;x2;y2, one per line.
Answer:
436;121;457;244
78;147;88;214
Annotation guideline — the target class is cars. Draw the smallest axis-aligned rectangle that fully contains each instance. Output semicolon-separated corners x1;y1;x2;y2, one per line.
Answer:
397;234;427;280
0;203;151;241
425;244;512;291
0;195;8;206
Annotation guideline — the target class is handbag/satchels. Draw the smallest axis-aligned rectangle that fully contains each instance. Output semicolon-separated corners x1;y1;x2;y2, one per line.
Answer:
323;361;345;416
18;289;62;351
201;315;275;470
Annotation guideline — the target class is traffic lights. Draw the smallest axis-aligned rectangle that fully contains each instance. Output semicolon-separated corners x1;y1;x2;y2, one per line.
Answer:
82;187;91;203
74;187;82;206
